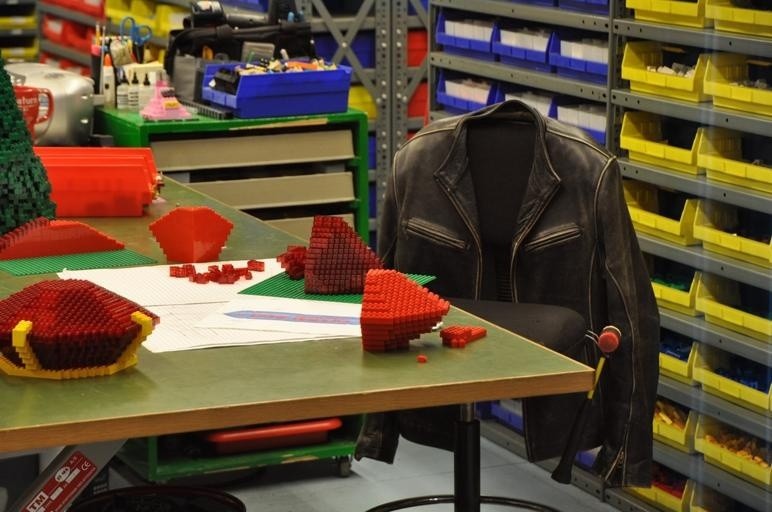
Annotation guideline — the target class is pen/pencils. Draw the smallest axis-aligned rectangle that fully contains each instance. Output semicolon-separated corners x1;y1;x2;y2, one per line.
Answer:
91;22;139;64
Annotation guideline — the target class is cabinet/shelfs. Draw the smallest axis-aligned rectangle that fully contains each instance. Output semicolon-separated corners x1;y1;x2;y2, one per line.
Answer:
424;3;770;512
3;4;430;263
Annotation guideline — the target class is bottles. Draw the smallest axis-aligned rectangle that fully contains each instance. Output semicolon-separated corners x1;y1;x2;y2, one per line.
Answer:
102;52;115;104
116;67;129;109
141;72;155;108
129;72;140;109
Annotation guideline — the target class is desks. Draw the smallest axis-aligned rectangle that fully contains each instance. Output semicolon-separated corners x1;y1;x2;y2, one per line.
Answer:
1;172;597;508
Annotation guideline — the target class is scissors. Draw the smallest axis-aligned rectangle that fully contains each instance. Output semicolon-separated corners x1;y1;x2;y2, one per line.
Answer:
120;17;152;63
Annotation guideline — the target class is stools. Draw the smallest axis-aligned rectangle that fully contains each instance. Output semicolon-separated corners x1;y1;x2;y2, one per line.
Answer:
365;114;619;511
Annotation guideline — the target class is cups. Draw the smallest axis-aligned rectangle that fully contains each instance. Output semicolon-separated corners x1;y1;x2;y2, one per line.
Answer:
13;85;55;140
